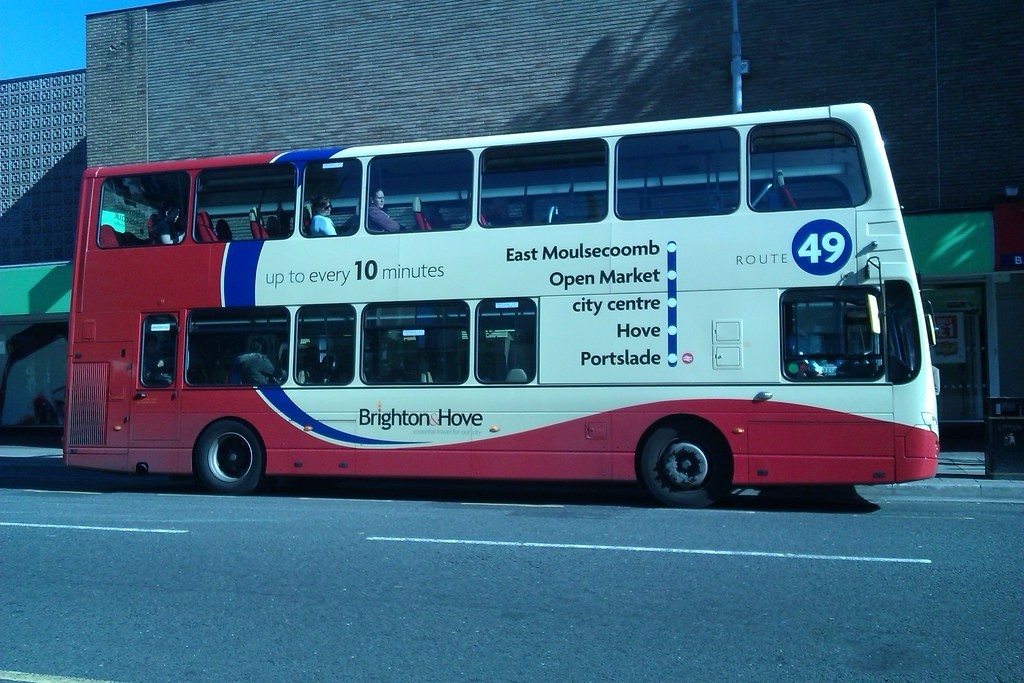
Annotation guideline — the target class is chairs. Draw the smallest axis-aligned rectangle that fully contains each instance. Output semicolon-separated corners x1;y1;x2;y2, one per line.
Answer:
198;208;268;242
303;202;314;236
481;203;496;227
412;197;448;231
99;214;159;248
775;169;797;208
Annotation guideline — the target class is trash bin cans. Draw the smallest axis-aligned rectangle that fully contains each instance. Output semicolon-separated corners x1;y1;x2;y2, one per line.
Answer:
983;396;1024;479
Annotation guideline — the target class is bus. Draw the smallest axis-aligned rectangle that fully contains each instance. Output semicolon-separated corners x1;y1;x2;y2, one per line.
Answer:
63;100;941;510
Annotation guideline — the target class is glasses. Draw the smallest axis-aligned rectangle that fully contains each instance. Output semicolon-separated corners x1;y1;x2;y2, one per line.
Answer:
324;205;332;210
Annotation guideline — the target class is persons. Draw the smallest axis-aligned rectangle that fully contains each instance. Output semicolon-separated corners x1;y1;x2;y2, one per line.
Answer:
235;337;276;385
155;204;186;243
368;189;400;232
787;317;838;377
309;193;337;237
488;195;515;226
